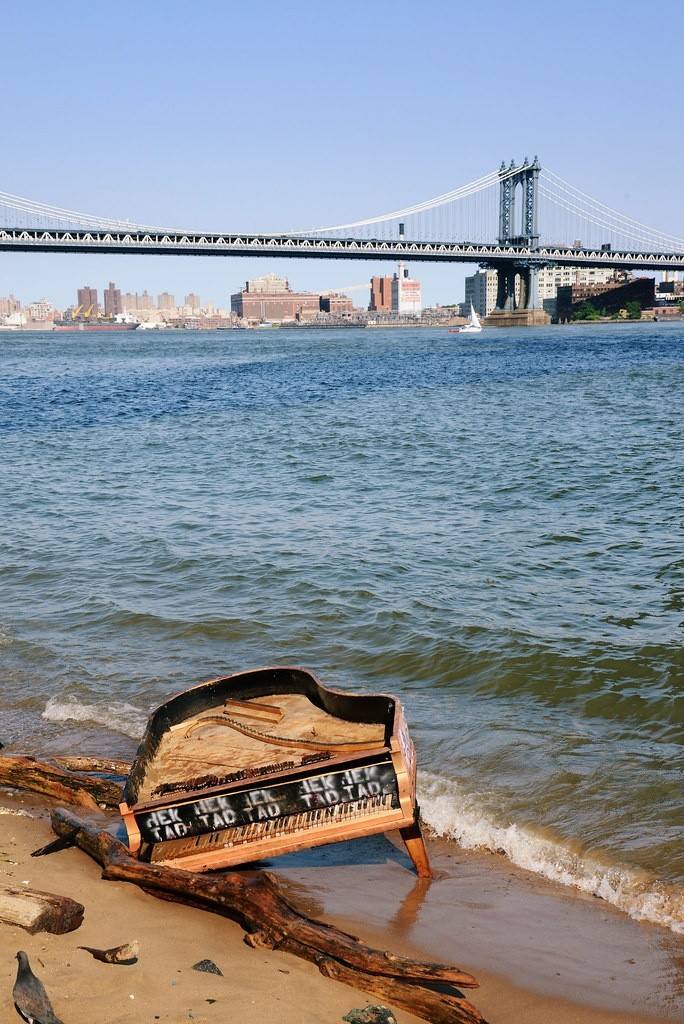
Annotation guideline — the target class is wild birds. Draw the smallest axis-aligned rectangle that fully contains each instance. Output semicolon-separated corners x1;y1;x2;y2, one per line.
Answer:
13;951;64;1024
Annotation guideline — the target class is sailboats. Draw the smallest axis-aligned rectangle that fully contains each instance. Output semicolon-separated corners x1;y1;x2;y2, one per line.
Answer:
457;304;483;334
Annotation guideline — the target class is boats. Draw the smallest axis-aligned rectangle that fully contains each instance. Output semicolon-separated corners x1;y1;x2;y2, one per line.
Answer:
53;319;140;331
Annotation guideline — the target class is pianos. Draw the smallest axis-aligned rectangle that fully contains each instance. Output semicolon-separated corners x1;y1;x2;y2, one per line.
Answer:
118;664;433;880
